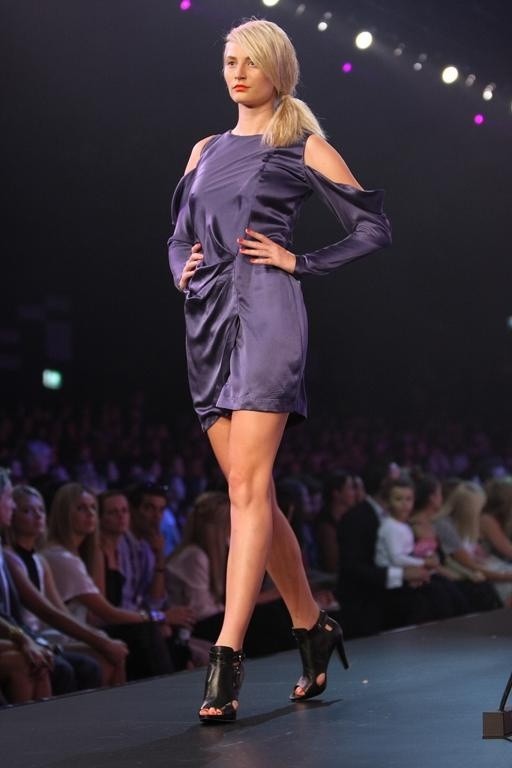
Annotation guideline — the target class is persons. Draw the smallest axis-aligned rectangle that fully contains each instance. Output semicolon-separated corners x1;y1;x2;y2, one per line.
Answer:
1;388;511;702
165;16;393;727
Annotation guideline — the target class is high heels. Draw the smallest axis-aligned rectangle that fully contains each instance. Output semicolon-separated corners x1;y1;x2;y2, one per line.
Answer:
289;612;350;701
198;647;243;726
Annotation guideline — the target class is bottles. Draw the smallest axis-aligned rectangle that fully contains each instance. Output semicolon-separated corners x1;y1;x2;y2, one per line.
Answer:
173;600;198;650
